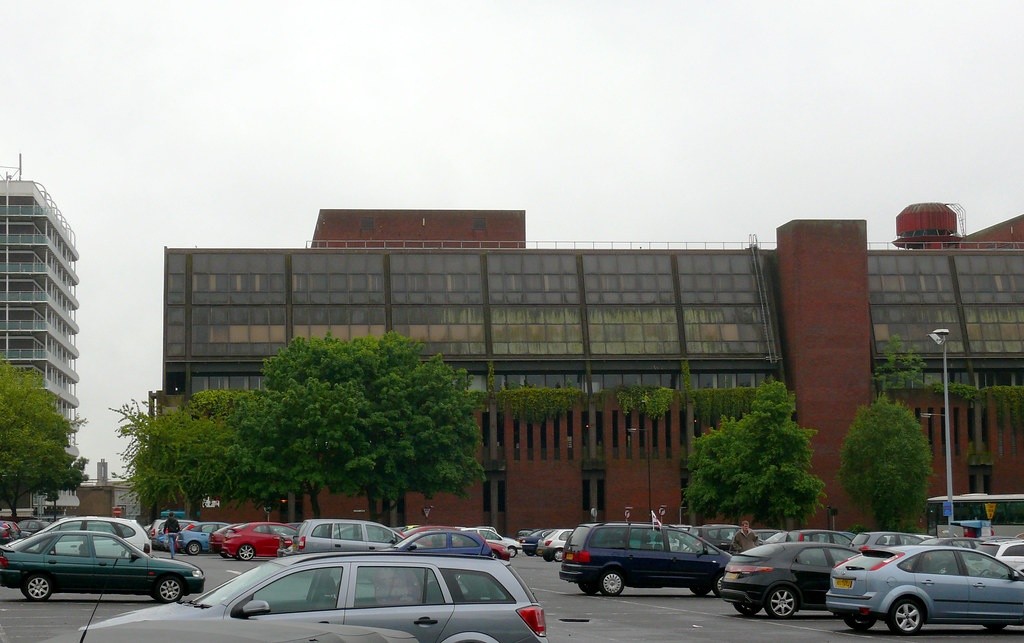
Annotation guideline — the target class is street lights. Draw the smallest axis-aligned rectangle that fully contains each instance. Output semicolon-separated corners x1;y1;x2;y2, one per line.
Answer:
926;328;955;525
626;428;653;524
920;412;955;525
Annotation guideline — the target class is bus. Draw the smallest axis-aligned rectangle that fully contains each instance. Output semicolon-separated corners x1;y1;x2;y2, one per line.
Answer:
925;492;1024;536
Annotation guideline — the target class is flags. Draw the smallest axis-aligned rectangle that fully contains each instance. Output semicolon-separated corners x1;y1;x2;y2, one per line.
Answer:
651;510;662;532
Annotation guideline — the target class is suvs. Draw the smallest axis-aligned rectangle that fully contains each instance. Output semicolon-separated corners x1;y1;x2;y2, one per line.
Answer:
41;550;549;643
0;515;153;557
556;520;733;599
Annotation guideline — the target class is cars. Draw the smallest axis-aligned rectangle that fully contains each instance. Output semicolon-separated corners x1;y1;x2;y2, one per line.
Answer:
825;544;1024;636
515;527;576;562
665;523;1024;577
0;530;206;605
720;542;862;621
284;518;433;554
144;519;299;561
382;524;523;560
0;519;50;544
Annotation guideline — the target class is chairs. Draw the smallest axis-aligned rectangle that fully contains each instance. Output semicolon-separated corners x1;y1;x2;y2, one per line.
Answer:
312;575;337;608
654;534;663;550
640;535;653;549
79;544;89;557
889;537;895;546
431;534;444;547
879;537;887;545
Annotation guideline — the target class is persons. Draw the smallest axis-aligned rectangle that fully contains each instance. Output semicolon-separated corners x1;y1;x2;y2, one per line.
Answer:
733;521;759;553
164;512;180;559
